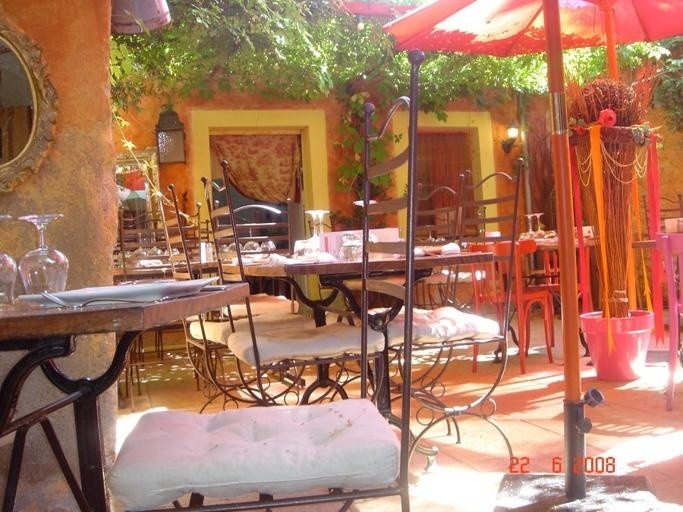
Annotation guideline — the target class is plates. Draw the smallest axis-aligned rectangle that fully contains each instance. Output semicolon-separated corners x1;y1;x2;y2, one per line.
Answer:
18;275;220;307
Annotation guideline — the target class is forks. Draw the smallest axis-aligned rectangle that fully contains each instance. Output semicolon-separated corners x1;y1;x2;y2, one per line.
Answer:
35;294;180;309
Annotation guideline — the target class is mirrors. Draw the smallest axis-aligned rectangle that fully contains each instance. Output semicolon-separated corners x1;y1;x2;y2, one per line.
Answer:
0;17;59;193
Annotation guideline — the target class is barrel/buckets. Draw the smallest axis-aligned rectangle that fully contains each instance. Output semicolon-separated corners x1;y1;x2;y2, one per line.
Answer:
580;309;652;380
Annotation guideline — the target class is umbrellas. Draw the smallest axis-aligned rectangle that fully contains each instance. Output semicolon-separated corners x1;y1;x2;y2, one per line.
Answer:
378;0;683;501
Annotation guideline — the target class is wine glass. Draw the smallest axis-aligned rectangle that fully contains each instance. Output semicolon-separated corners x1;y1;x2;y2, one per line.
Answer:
304;210;335;262
523;213;545;240
0;213;70;311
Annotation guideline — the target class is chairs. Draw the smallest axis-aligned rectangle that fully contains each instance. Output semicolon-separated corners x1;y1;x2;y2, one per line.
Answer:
0;49;683;512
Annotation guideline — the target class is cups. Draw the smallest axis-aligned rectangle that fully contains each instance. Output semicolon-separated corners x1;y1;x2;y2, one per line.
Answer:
199;242;214;263
228;240;277;253
665;218;678;234
118;248;179;267
292;240;309;259
